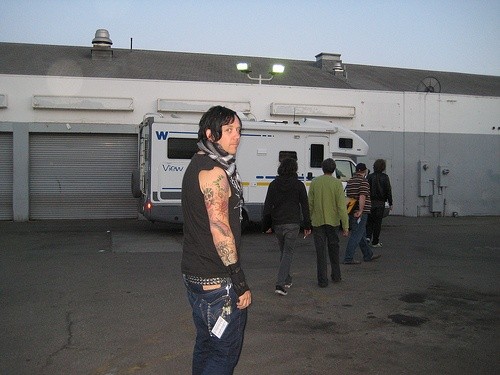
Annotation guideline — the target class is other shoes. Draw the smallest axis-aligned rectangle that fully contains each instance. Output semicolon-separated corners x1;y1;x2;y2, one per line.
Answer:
285;282;293;288
372;243;383;248
319;278;328;287
366;238;370;243
343;258;361;264
363;254;381;262
331;275;342;282
275;286;288;296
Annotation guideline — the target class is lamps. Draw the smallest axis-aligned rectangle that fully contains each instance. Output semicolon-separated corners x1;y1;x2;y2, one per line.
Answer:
236;62;285;84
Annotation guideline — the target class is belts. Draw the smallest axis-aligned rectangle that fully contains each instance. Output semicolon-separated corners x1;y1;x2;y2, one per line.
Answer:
185;275;227;286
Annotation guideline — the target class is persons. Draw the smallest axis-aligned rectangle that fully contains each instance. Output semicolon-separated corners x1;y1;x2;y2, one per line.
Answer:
308;157;350;288
365;158;393;248
263;157;311;296
343;162;381;265
181;104;252;375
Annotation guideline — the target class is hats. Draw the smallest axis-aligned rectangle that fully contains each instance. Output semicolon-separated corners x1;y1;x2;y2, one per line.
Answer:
356;163;367;172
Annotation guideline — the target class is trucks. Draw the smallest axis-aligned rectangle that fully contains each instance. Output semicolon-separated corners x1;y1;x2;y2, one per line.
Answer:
132;113;371;232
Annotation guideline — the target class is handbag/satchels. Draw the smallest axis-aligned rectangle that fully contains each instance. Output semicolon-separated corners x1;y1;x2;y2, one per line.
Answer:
345;196;357;215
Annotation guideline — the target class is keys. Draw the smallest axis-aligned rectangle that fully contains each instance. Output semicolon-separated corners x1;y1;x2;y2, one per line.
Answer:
221;294;233;323
